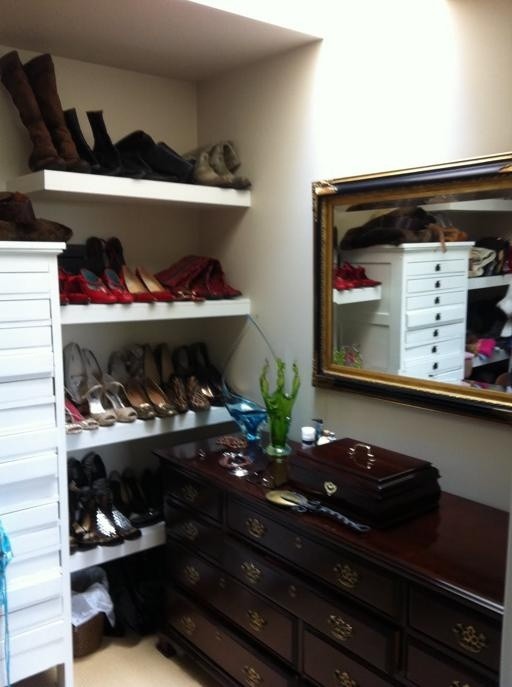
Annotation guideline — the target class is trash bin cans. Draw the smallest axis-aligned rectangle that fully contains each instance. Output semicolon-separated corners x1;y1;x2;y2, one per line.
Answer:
71;568;109;657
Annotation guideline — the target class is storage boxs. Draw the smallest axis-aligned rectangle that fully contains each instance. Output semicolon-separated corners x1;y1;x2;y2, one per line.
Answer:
285;435;444;531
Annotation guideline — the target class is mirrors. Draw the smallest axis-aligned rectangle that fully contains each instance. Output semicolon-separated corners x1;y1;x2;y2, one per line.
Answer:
310;151;512;424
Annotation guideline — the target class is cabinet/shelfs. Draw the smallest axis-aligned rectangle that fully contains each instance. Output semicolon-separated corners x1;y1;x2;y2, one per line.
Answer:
0;239;75;687
6;168;252;574
468;273;511;368
337;240;476;384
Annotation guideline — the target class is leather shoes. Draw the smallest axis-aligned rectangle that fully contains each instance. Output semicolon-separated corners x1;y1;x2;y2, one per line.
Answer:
65;340;237;434
67;452;166;553
59;237;174;304
333;261;383;291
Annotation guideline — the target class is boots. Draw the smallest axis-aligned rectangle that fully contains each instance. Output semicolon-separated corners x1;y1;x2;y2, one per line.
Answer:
155;253;244;301
2;49;250;188
101;562;161;640
340;204;470;249
469;237;512;277
0;191;74;244
